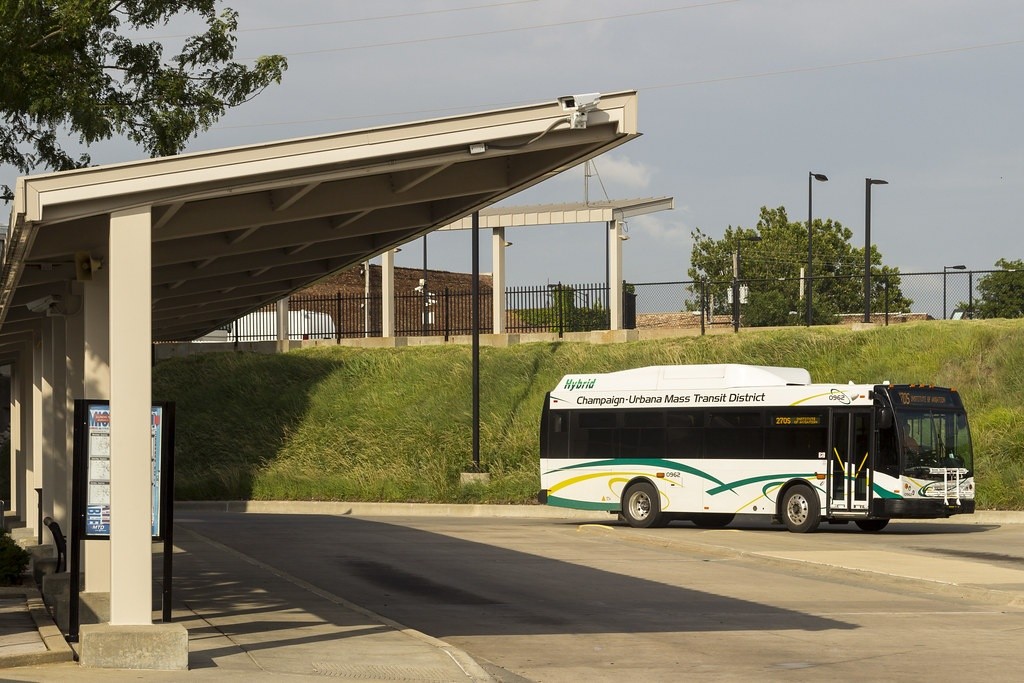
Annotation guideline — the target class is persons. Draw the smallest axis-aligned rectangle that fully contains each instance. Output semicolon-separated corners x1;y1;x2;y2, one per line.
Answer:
901;425;918;449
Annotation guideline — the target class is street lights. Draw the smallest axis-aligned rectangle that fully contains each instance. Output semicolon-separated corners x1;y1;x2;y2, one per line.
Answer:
943;265;966;321
734;237;763;333
862;178;889;324
806;171;829;328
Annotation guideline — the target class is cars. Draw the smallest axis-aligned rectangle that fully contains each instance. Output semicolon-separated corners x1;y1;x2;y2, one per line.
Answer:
949;309;975;322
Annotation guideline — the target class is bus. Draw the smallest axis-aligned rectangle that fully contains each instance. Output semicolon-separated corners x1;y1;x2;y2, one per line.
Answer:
537;363;976;533
